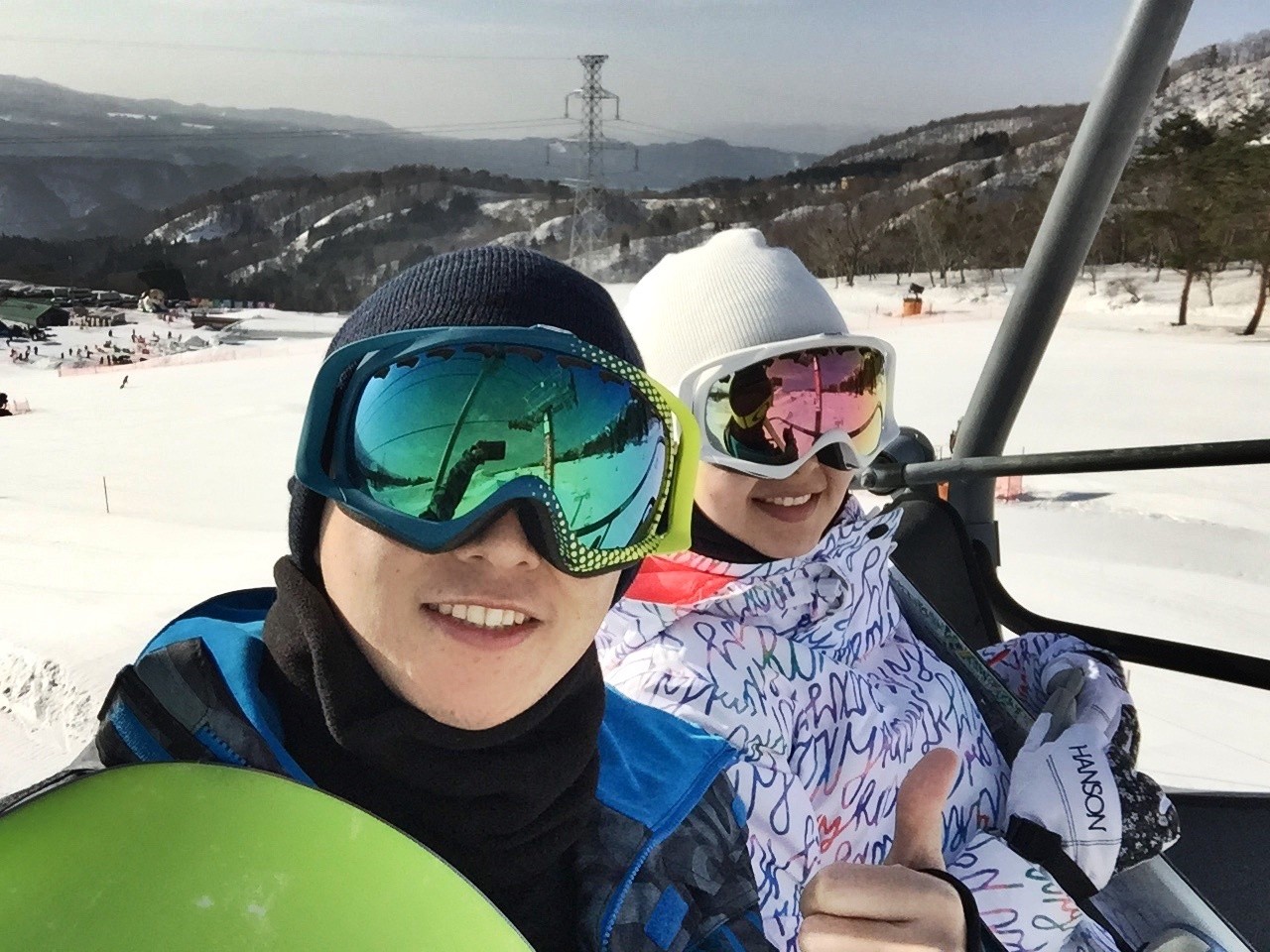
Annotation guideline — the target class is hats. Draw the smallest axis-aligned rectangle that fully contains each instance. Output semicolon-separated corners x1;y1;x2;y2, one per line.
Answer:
287;246;644;606
622;226;850;408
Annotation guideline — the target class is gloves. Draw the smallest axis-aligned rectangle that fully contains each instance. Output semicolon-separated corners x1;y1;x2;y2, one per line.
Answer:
1040;651;1141;767
1000;686;1123;899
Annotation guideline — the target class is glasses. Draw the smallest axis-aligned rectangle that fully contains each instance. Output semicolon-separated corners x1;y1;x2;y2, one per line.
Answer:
679;334;901;482
292;325;700;578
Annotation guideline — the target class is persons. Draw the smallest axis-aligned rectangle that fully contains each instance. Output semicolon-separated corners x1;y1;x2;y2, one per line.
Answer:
6;296;207;366
420;442;487;521
597;227;1182;952
0;244;1009;952
723;366;799;467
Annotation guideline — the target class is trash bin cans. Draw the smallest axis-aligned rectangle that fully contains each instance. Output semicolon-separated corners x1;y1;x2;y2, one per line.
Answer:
904;297;922;315
